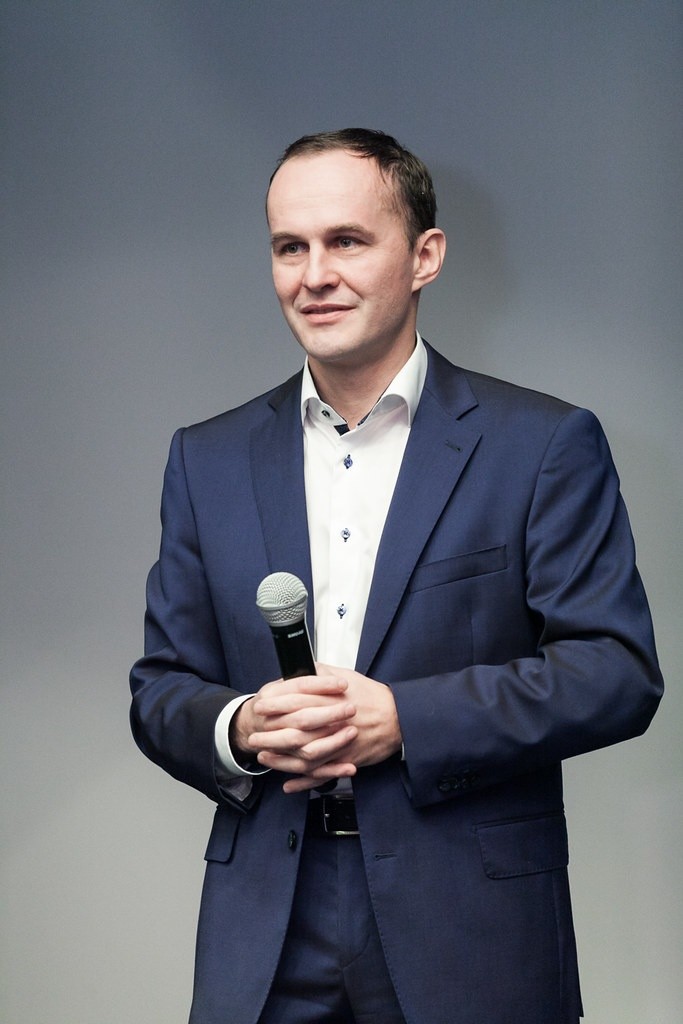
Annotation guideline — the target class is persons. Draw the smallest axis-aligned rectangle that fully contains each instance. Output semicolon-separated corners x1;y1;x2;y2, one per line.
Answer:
127;128;663;1024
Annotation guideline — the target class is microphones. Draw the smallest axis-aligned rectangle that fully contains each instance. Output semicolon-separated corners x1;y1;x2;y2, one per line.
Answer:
256;571;339;792
